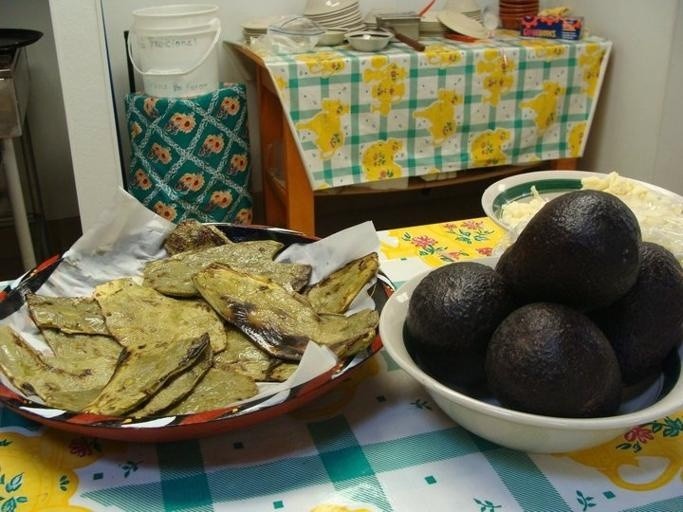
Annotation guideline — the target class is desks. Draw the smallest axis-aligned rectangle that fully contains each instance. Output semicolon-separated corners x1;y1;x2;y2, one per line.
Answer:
221;20;613;238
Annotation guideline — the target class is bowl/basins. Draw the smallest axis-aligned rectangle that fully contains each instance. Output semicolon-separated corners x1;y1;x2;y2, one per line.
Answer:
349;32;391;51
482;171;683;259
378;253;682;455
500;0;538;29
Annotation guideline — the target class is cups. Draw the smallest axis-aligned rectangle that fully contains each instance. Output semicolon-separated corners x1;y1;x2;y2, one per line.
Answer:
320;32;349;46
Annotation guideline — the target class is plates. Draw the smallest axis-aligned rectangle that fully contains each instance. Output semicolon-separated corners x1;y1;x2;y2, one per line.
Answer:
304;0;366;30
439;11;489;40
347;29;367;32
241;18;267;43
0;223;395;444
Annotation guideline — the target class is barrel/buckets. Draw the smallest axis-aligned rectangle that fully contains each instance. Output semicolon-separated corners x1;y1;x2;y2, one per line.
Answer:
128;4;222;99
